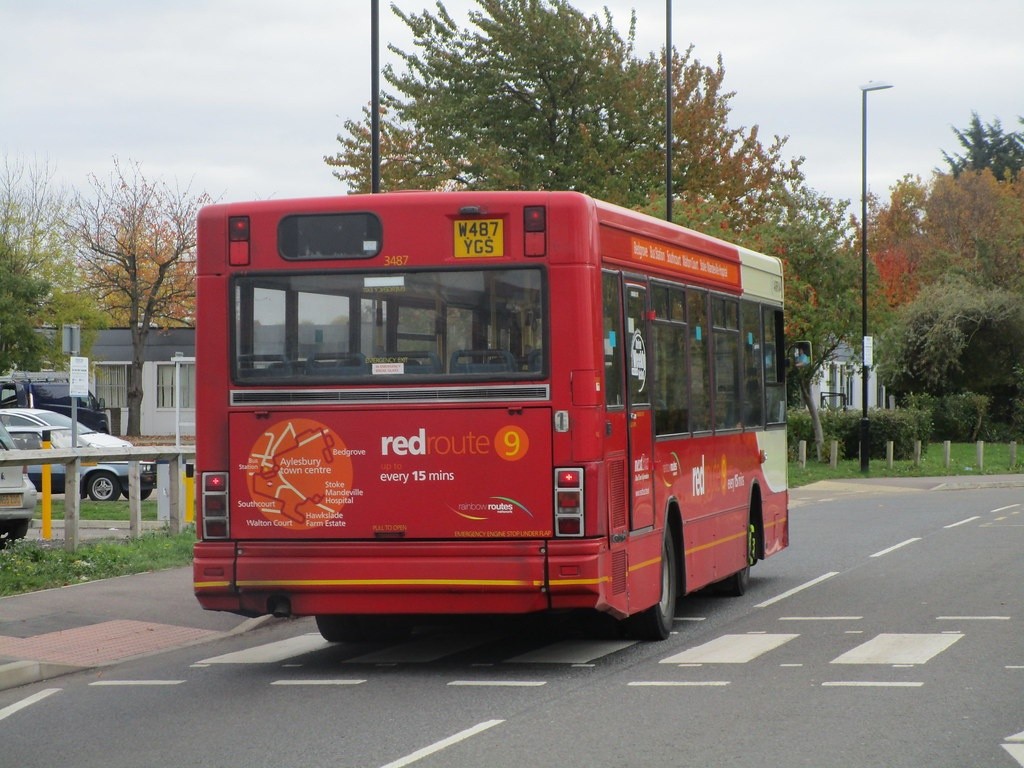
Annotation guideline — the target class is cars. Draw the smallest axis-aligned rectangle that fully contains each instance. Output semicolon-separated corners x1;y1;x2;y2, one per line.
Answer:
0;407;159;502
0;437;37;539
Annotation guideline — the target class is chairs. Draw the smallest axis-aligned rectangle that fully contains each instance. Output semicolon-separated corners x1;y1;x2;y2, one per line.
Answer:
236;350;541;374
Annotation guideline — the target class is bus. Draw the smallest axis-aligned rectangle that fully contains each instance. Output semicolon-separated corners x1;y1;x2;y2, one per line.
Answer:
195;191;816;642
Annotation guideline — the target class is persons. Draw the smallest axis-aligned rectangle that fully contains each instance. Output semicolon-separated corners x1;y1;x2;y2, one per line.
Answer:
796;347;808;366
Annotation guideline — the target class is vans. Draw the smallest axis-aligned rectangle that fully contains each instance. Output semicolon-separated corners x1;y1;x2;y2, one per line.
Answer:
0;376;109;436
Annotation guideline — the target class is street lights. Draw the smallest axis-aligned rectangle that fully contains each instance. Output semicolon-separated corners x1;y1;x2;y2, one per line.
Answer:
172;356;185;447
860;80;897;471
